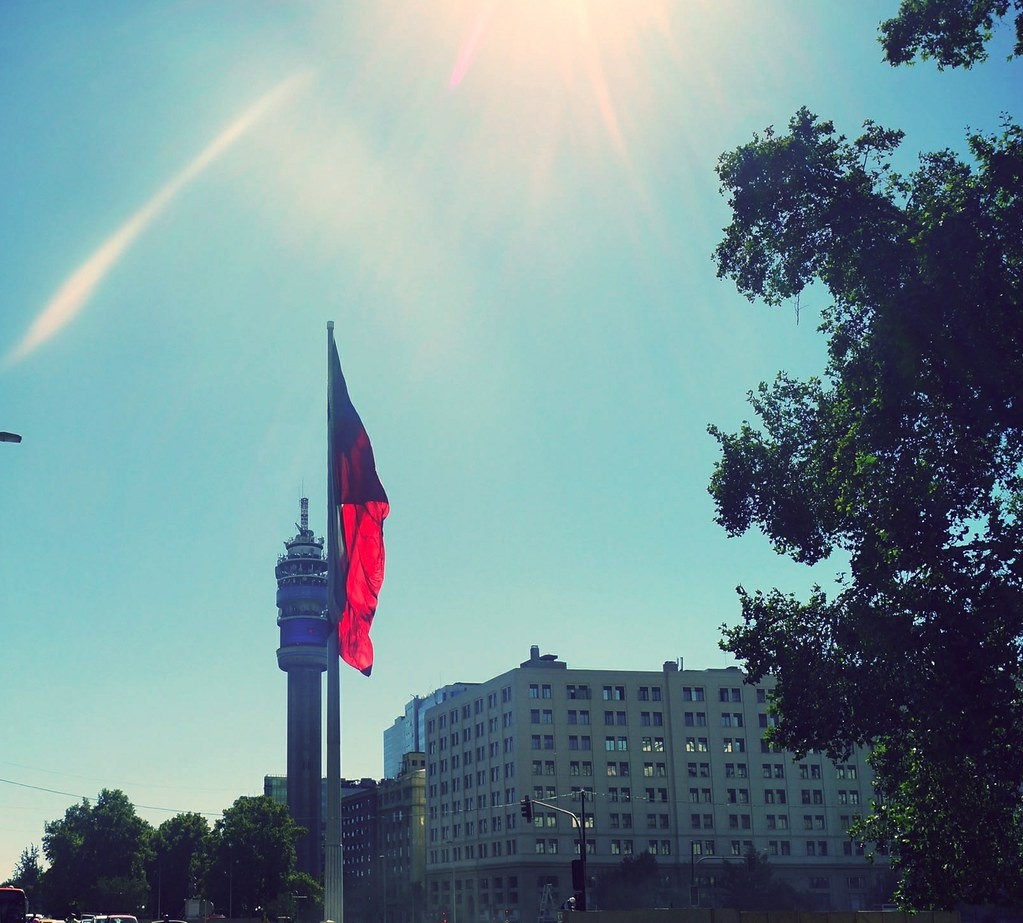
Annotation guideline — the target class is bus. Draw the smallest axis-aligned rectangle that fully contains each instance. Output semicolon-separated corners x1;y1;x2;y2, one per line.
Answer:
0;885;30;923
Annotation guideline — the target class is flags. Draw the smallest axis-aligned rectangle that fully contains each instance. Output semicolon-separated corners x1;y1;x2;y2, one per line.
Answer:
330;343;389;677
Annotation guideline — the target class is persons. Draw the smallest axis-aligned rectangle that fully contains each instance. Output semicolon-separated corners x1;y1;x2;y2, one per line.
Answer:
25;913;42;923
65;913;80;923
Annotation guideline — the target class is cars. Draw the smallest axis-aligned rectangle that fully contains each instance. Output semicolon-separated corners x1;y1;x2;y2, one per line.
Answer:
65;913;138;923
28;917;53;923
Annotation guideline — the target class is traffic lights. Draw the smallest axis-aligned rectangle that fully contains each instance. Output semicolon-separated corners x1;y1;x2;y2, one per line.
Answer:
520;795;531;823
